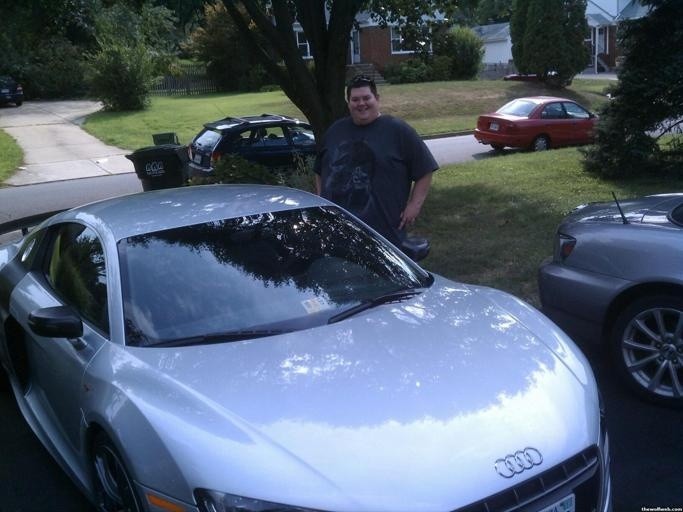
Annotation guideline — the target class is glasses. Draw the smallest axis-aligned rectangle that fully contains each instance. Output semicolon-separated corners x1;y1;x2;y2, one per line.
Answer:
352;76;373;82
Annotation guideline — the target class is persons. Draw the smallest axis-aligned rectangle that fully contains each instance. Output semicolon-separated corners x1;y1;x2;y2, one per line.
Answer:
312;74;440;252
257;128;267;140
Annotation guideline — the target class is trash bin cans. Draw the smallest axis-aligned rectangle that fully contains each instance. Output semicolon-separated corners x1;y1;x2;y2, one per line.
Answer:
125;133;189;193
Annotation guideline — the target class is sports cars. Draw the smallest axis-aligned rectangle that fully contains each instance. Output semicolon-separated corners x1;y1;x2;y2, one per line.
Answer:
0;176;623;512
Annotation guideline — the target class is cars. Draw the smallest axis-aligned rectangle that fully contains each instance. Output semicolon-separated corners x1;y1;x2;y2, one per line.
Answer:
471;94;601;152
534;186;682;412
0;71;24;109
501;69;556;82
182;111;317;180
